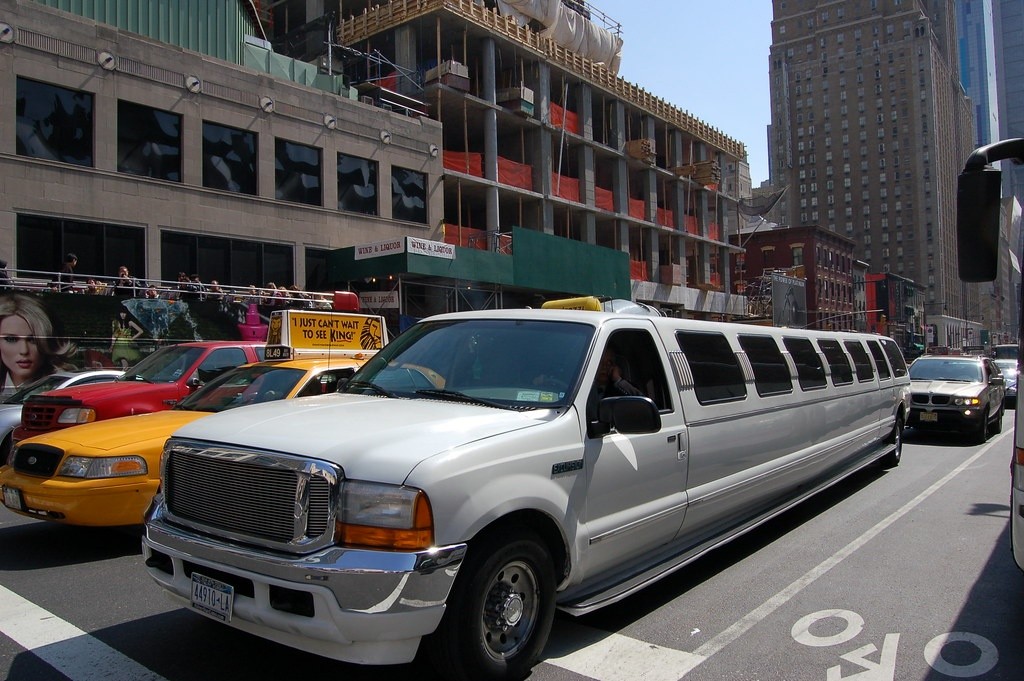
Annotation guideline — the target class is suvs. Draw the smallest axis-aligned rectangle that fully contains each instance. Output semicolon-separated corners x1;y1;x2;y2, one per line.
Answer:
8;340;331;465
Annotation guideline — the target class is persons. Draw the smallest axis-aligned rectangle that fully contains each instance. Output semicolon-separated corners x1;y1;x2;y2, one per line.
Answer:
87;267;332;309
0;289;78;395
532;343;644;419
50;253;77;293
109;306;143;371
0;260;15;288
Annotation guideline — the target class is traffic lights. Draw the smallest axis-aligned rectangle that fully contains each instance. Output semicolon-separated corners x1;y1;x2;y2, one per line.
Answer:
880;314;887;326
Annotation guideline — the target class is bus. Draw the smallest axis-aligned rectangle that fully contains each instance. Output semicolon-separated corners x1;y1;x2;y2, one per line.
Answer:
956;136;1023;574
925;346;961;355
992;344;1019;359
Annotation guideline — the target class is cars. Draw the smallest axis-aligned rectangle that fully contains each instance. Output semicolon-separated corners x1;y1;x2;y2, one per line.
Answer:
0;309;448;526
905;353;1004;444
993;358;1017;397
1;369;126;468
140;306;913;681
